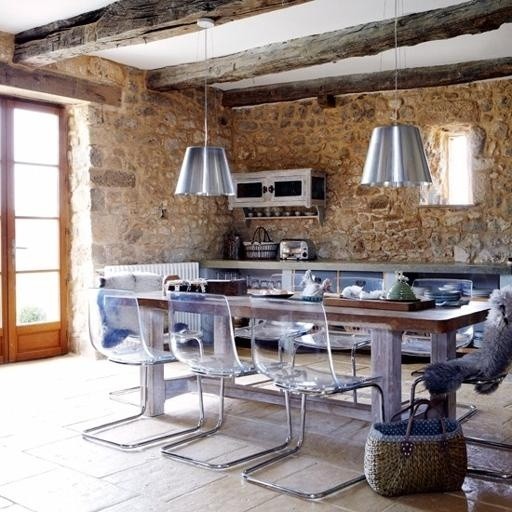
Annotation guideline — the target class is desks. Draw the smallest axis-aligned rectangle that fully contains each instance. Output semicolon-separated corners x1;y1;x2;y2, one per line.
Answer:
106;288;490;422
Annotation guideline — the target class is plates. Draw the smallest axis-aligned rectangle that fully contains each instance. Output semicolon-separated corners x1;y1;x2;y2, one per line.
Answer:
252;291;295;304
304;211;315;215
424;282;493;302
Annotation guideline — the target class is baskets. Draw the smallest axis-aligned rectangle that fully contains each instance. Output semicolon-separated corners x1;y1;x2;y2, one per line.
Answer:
364;377;467;495
243;227;278;260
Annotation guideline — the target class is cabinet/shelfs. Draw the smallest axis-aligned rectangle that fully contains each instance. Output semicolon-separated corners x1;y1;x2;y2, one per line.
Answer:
225;167;327;210
200;256;512;358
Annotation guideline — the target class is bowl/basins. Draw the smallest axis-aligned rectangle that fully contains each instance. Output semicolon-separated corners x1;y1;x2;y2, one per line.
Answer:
413;286;424;295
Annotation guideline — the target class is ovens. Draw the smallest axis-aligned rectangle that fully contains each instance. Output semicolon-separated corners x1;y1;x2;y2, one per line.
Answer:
279;238;309;260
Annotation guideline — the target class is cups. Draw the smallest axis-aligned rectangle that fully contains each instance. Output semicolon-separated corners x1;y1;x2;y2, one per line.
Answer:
250;278;282;290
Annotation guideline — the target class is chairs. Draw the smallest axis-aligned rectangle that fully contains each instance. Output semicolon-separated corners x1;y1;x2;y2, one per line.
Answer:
294;277;385;403
231;273;319;392
400;279;478;422
240;297;385;500
81;289;205;450
408;285;512;485
98;274;185;410
160;289;293;473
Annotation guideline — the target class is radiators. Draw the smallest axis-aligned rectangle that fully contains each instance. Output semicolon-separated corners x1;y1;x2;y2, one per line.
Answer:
105;261;200;346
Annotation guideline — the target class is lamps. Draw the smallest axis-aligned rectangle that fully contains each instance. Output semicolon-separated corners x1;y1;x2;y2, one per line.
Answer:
358;0;432;189
173;17;236;198
